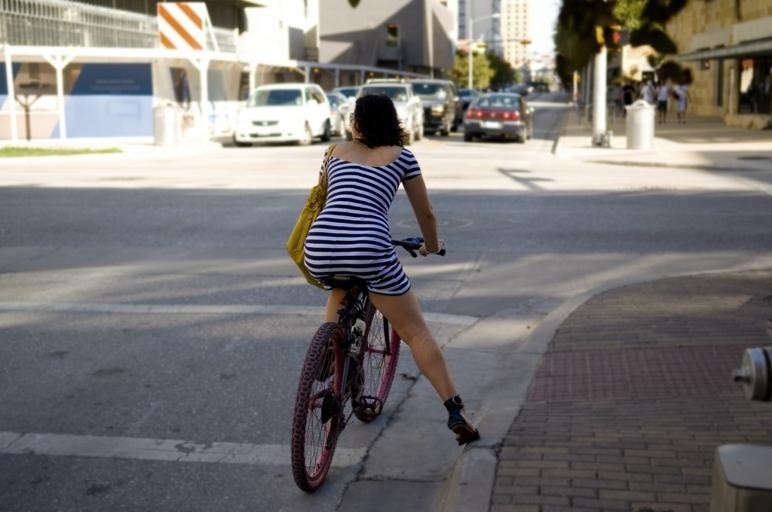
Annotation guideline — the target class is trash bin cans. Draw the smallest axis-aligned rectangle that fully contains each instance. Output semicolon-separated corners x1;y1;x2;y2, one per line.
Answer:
624;99;657;150
154;105;179;145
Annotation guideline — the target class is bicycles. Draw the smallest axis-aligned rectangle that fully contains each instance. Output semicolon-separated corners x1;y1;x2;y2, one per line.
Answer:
289;234;448;494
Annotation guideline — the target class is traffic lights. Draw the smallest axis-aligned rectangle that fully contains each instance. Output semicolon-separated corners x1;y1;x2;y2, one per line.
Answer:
593;27;603;43
610;28;627;46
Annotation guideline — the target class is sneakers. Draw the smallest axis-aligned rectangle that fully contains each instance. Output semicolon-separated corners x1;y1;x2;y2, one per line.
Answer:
315;350;337;380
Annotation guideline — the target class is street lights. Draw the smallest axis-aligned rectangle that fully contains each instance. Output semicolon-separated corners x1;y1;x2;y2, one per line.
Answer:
467;13;502;88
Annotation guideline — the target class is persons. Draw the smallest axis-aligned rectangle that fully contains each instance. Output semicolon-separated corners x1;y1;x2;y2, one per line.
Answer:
605;76;693;126
303;93;481;446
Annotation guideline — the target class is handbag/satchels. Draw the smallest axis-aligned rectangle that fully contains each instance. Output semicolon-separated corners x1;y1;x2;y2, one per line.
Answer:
284;182;332;294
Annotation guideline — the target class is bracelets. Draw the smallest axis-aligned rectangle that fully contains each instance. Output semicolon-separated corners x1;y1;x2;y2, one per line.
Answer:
423;242;442;254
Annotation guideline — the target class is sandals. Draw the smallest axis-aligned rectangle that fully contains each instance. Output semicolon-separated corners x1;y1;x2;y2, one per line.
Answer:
446;412;480;446
